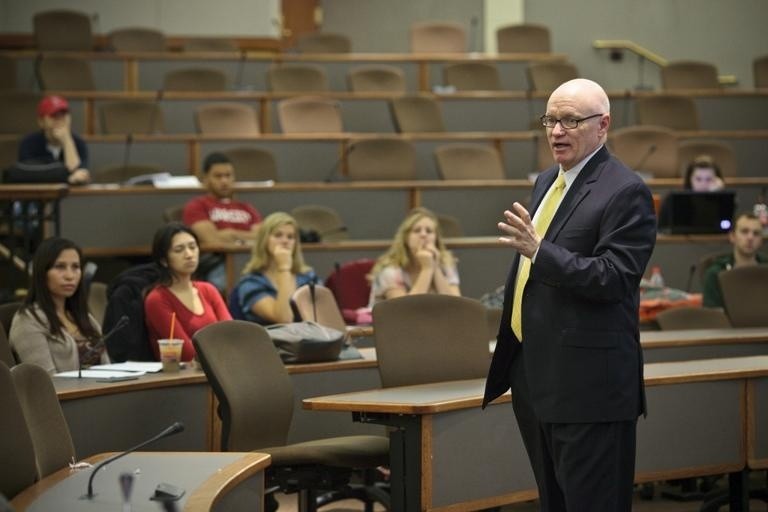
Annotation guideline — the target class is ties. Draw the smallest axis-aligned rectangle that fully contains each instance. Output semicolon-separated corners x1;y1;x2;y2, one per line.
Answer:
510;174;565;343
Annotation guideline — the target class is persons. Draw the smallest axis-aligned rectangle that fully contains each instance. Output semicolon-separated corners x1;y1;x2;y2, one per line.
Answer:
226;211;324;326
10;235;112;376
700;212;767;309
481;77;655;511
180;150;264;243
366;206;461;311
17;95;88;186
142;223;233;362
680;156;724;194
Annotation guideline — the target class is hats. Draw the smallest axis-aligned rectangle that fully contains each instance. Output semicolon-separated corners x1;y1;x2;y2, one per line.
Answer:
36;96;68;116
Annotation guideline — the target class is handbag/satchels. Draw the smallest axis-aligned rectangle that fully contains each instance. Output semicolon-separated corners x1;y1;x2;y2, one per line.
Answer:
264;321;343;363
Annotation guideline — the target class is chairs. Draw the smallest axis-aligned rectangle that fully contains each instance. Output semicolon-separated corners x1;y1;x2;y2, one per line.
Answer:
2;363;78;502
371;294;501;441
191;318;393;505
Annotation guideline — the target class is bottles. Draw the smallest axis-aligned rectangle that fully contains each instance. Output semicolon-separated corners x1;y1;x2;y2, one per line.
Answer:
650;266;664;287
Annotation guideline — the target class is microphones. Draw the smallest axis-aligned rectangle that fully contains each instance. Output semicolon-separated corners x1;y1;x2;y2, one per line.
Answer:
325;143;358;182
622;89;631;129
149;89;163;134
687;264;697;293
525;89;534;131
300;226;348;243
92;13;106;52
635;54;655;91
78;315;130;378
238;54;246;89
632;144;658;172
334;262;340;306
308;279;317;323
88;421;185;498
470;16;480;52
532;135;540;172
119;134;133;186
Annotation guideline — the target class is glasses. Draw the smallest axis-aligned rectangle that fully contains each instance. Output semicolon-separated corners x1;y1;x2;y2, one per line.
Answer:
540;114;602;129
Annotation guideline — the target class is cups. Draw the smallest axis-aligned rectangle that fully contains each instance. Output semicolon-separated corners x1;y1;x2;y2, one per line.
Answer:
157;339;184;372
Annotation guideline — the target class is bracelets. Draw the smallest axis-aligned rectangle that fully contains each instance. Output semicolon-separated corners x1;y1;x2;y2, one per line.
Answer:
277;268;291;273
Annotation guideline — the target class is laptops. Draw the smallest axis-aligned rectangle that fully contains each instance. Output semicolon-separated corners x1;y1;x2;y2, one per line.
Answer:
660;190;737;237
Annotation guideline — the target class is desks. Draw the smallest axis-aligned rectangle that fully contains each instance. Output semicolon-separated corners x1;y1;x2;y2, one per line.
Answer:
2;446;276;511
56;327;768;457
303;352;768;510
